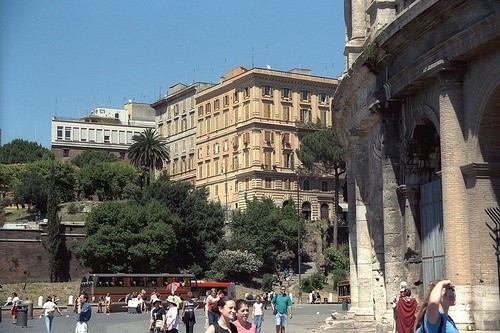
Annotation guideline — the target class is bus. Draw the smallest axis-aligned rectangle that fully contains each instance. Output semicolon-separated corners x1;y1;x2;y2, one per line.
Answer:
336;280;351;305
79;273;199;306
190;280;236;305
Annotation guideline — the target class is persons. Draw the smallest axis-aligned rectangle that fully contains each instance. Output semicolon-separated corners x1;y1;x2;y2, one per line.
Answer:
149;288;181;333
252;295;264;333
125;289;147;313
52;295;63;316
203;288;257;333
311;288;322;304
97;293;111;313
415;280;460;333
180;292;199;333
271;286;292;333
392;282;418;333
75;294;91;333
11;292;19;324
245;289;276;310
40;296;63;333
284;268;291;283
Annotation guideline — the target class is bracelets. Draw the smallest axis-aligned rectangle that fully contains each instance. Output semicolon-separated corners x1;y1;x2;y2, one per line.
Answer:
77;303;81;306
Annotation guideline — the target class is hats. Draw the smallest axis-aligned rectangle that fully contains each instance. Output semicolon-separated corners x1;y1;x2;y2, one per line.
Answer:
165;296;178;306
153;297;160;304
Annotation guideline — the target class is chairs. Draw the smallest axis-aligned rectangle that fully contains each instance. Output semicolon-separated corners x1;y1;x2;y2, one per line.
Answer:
81;280;169;287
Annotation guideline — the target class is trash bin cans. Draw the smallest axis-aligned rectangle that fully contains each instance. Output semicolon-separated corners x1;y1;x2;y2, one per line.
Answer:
342;300;348;310
324;298;328;304
24;300;34;320
16;304;28;327
